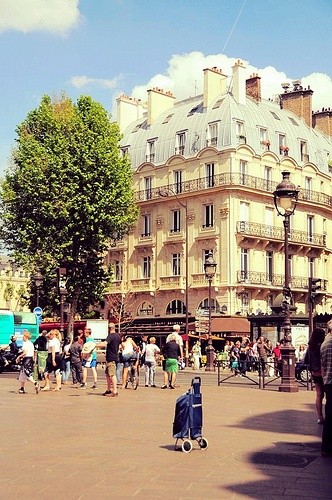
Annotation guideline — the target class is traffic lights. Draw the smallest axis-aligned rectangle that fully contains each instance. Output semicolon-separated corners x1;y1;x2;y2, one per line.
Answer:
309;277;323;302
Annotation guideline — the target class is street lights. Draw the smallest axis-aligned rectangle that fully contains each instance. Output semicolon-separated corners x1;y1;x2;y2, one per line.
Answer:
56;267;68;340
157;186;189;368
33;271;42;310
203;252;218;372
273;169;302;393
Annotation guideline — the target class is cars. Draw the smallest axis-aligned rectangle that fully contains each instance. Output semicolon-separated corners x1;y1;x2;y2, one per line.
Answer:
295;358;315;384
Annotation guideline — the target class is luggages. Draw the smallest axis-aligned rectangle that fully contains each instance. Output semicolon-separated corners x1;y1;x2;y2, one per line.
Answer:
172;376;208;453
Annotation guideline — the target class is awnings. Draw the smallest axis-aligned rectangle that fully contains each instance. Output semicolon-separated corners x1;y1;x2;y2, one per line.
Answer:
119;316;195;337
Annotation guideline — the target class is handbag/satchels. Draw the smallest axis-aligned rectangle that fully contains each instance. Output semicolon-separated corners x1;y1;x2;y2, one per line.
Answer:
140;353;145;366
231;357;238;367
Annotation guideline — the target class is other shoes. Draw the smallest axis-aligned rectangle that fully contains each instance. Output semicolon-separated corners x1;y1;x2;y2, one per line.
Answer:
316;418;323;423
102;390;111;395
78;382;87;389
35;382;40;394
170;384;174;388
160;383;167;388
90;384;98;390
18;389;24;393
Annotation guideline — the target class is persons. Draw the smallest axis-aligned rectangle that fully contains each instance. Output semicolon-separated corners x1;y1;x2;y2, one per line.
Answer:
166;324;184;388
1;336;17;366
33;329;98;391
138;336;147;368
224;336;281;377
145;337;160;387
121;335;139;390
320;320;332;461
304;328;325;424
161;336;182;389
14;332;40;394
33;329;48;383
298;345;306;361
192;340;203;370
95;323;123;399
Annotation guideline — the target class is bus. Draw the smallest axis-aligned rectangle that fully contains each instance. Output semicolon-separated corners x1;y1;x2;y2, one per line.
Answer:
38;319;110;352
0;308;40;353
